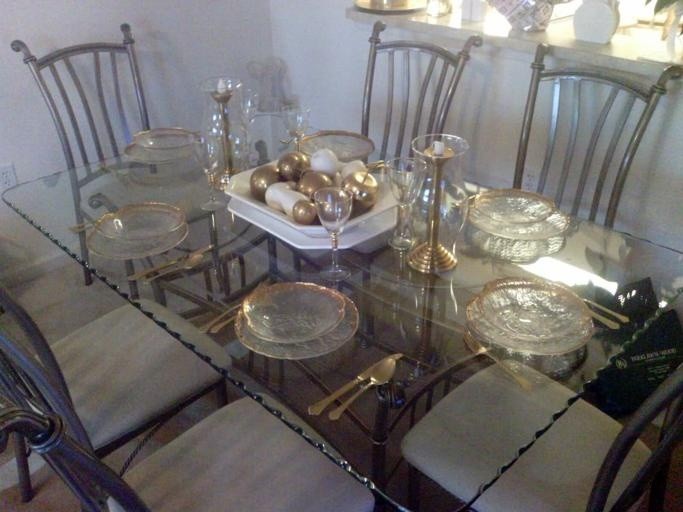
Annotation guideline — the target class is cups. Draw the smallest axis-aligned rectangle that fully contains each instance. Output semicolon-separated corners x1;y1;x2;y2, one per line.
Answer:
409;132;470;245
400;278;458;383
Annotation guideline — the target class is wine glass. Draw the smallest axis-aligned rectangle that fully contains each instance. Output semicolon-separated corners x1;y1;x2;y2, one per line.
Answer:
281;105;311;152
191;132;227;212
314;187;353;282
386;158;427;251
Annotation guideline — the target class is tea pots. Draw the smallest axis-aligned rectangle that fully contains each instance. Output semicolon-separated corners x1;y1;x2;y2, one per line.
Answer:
188;77;260;179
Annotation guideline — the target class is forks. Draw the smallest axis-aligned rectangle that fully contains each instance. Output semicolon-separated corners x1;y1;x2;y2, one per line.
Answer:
197;278;276;334
465;333;532;391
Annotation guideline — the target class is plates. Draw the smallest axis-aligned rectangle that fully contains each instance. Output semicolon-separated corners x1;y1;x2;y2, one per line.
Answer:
123;127;198;164
86;202;187;260
466;278;594;355
462;189;570;240
235;283;359;360
354;0;428;13
297;129;374;160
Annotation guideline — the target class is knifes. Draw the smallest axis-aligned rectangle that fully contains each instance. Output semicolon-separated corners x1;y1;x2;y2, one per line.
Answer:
307;352;404;416
495;261;628;329
127;243;216;281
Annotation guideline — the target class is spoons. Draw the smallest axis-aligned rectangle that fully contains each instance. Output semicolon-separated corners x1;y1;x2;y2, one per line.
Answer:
329;358;396;421
144;254;204;282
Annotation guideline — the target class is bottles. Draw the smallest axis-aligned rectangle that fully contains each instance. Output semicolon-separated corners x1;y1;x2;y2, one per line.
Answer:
243;54;292;168
